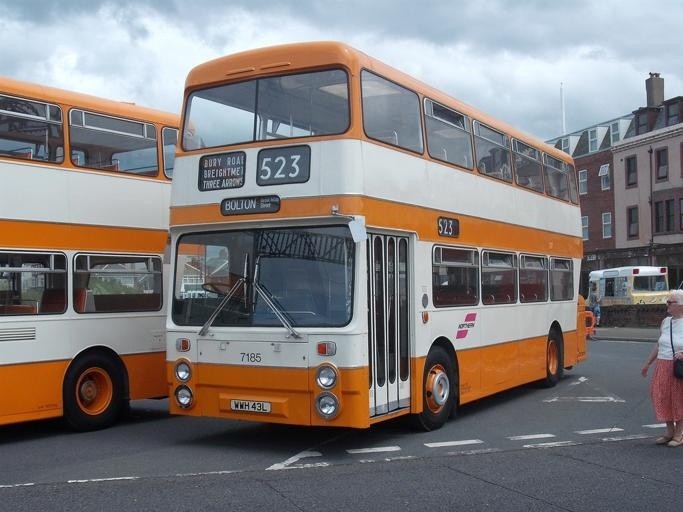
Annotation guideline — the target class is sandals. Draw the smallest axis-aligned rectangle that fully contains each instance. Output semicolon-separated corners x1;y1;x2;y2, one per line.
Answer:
656;434;683;447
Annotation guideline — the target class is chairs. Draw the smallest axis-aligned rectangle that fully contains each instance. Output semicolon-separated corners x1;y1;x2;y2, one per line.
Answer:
432;281;562;314
367;126;577;202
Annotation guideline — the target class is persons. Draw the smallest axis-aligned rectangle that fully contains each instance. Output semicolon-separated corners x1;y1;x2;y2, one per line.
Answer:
640;289;682;448
587;286;600;326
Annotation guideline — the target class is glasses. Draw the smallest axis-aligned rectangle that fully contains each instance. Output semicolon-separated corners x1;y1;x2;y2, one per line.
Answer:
667;300;678;305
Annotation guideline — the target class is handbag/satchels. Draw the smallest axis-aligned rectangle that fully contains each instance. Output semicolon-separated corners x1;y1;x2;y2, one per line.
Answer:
673;358;683;380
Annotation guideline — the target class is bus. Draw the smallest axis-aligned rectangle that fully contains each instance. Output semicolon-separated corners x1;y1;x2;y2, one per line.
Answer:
158;38;595;436
0;73;211;444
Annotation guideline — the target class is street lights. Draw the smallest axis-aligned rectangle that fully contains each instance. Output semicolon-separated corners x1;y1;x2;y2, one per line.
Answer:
646;144;657;266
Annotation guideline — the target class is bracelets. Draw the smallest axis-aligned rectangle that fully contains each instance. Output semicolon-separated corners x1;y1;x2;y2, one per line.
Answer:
647;361;651;365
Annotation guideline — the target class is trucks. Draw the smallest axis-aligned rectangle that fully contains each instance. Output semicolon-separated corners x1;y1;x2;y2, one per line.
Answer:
584;263;674;305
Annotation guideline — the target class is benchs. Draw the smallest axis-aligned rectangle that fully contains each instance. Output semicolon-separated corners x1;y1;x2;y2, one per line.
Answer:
1;146;162;182
0;284;166;317
